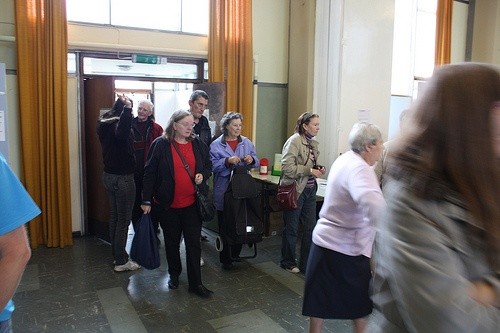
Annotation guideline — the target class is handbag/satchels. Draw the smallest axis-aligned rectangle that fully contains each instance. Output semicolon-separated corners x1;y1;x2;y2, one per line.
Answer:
196;190;217;221
276;181;297;209
130;212;160;270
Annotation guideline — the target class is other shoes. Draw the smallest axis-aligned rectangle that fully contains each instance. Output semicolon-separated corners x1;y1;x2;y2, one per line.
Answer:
190;284;213;297
114;257;143;271
200;257;204;266
232;256;242;261
168;278;179;289
224;261;233;269
287;265;300;273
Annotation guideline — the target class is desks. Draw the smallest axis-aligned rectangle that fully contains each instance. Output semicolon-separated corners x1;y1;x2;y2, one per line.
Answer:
250;170;327;236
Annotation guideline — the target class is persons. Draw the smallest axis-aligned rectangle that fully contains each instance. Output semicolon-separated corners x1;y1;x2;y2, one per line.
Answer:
209;111;259;271
280;112;326;275
96;94;142;272
0;153;42;333
302;121;387;333
372;63;500;333
141;109;214;298
134;100;164;246
189;90;211;266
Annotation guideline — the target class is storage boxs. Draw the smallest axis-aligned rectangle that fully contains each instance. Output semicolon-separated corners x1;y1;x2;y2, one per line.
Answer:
265;208;284;236
272;165;283;175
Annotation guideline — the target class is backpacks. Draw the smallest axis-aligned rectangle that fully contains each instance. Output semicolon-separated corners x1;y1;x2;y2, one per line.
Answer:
224;158;264;247
